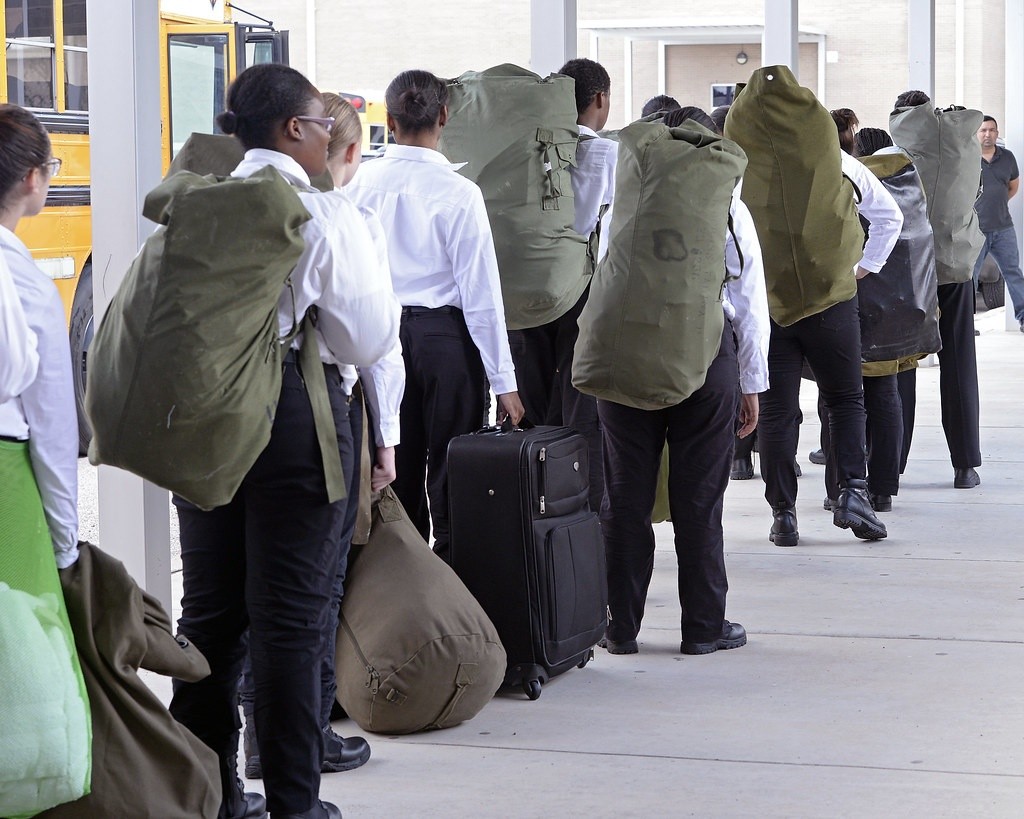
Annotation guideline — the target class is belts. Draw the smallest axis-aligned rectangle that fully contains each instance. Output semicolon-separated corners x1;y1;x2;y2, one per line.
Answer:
401;304;452;315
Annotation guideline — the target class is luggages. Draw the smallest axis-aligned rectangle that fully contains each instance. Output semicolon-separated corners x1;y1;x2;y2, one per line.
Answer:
446;412;611;697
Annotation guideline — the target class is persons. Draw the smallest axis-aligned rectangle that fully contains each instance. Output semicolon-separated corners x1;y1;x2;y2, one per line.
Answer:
168;62;405;819
346;70;525;543
557;57;982;655
0;102;79;569
973;115;1024;336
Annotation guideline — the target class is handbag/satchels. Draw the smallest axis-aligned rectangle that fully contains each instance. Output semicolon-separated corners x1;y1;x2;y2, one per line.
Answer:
334;483;507;733
33;541;223;818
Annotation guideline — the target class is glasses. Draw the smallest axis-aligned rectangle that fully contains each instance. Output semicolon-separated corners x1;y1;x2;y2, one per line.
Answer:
284;117;334;132
21;158;61;182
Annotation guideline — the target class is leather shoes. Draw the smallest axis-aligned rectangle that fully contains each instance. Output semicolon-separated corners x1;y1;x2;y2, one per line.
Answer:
730;458;754;479
769;507;799;546
954;467;980;488
833;487;887;539
216;754;267;819
274;797;343;819
240;700;265;780
319;684;371;772
596;632;639;654
823;494;838;512
867;489;892;512
680;619;747;654
809;449;826;464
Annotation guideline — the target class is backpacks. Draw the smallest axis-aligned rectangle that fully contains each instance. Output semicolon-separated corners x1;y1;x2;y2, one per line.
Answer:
436;63;611;331
888;105;986;284
722;64;864;328
573;120;744;410
856;153;943;377
85;164;346;512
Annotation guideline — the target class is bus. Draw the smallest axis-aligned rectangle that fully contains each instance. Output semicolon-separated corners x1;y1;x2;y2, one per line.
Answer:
2;0;304;459
